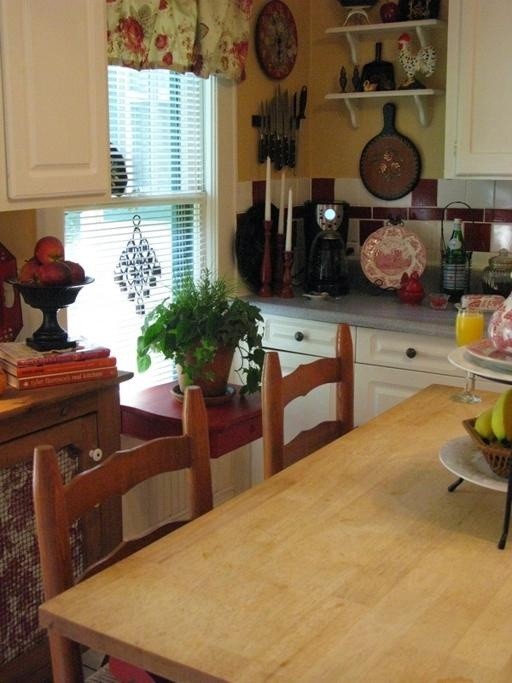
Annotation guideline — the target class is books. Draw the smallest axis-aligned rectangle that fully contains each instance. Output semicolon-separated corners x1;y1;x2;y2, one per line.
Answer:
0;338;111;369
0;354;117;377
4;363;118;391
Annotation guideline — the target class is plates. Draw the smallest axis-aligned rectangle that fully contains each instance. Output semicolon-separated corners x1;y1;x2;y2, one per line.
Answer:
438;434;510;493
170;384;236;405
359;224;427;291
447;339;511;381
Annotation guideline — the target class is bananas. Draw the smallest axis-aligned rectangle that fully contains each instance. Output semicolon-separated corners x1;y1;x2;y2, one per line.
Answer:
472;388;512;447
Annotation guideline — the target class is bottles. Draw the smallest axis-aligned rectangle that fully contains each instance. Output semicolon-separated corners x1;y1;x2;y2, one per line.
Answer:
339;65;358;91
442;216;470;305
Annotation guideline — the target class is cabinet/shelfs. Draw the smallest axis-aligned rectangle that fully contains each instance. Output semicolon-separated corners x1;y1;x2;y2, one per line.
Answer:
254;311;356;444
356;327;512;428
0;1;111;212
0;369;124;682
324;19;445;127
442;1;512;182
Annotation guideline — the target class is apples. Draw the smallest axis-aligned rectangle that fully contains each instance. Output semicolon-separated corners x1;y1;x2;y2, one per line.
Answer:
19;236;85;287
380;1;399;23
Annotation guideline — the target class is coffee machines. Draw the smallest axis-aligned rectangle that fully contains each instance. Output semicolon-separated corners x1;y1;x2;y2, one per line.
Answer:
301;197;353;302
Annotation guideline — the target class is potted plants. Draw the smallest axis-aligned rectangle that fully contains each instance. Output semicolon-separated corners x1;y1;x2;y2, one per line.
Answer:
135;267;264;407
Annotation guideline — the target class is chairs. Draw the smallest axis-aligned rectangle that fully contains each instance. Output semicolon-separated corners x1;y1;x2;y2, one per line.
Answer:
260;322;359;487
32;386;213;682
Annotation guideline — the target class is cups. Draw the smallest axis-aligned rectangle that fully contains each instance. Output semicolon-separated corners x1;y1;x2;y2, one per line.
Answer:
428;293;449;311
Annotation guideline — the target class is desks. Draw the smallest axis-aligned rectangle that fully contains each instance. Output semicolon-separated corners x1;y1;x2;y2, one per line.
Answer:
118;382;263;459
39;383;512;683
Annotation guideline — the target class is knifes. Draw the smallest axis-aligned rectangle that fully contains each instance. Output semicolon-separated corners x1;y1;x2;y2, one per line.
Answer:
258;83;296;169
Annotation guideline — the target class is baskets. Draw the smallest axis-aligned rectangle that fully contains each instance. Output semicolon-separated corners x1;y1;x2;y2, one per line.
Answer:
460;418;512;482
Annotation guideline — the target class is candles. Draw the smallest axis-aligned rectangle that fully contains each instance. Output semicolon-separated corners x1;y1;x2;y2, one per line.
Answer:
265;157;293;253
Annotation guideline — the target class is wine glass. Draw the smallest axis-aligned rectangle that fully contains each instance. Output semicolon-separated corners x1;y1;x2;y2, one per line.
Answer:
4;275;95;340
453;305;485;404
